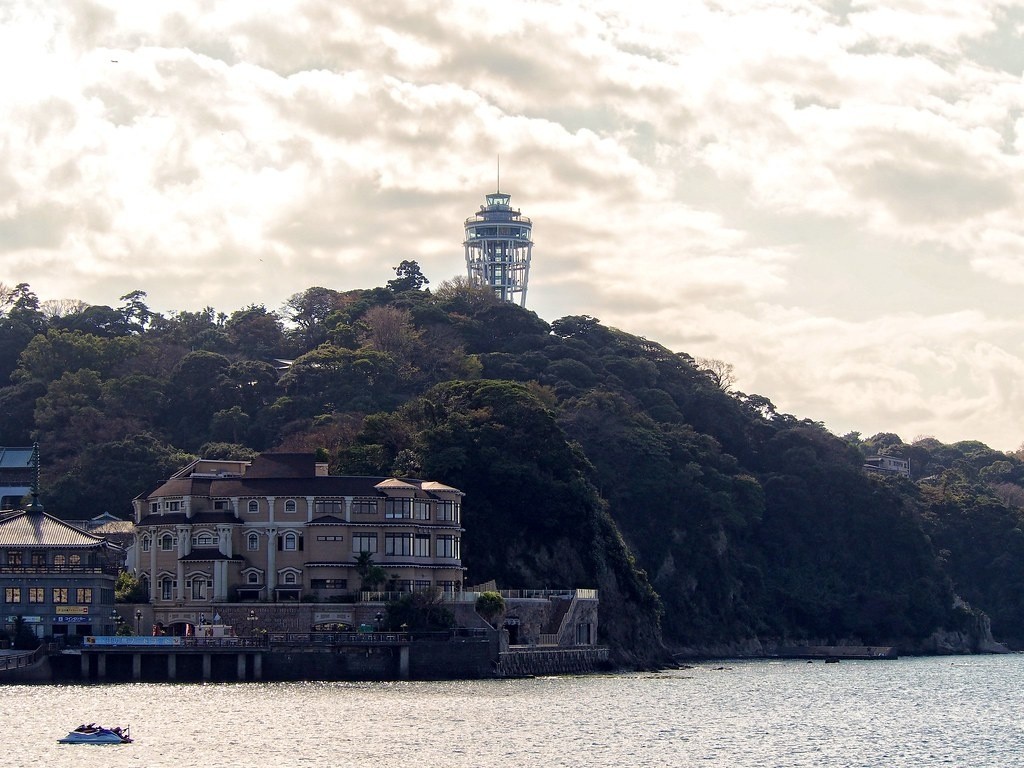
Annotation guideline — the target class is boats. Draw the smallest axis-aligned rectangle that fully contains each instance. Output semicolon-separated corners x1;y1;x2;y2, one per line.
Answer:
56;722;134;745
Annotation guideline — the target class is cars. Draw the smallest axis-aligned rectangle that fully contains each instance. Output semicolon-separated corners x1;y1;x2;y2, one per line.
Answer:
81;639;106;648
194;639;206;648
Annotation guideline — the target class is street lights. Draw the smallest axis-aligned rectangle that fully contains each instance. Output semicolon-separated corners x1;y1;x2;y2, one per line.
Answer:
374;610;383;631
246;610;258;645
136;610;141;635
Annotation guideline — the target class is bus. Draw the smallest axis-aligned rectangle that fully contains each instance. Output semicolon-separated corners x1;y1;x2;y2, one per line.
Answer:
449;628;489;644
